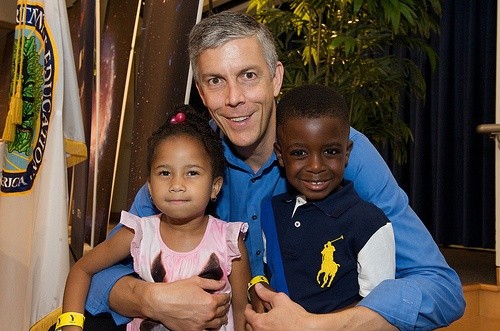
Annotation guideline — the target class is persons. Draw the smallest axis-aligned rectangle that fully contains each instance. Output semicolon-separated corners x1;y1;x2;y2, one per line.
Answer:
50;102;252;331
226;83;397;331
72;9;468;331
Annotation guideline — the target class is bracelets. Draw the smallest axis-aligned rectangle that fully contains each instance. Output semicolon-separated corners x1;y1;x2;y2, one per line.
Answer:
246;275;271;301
56;311;85;330
